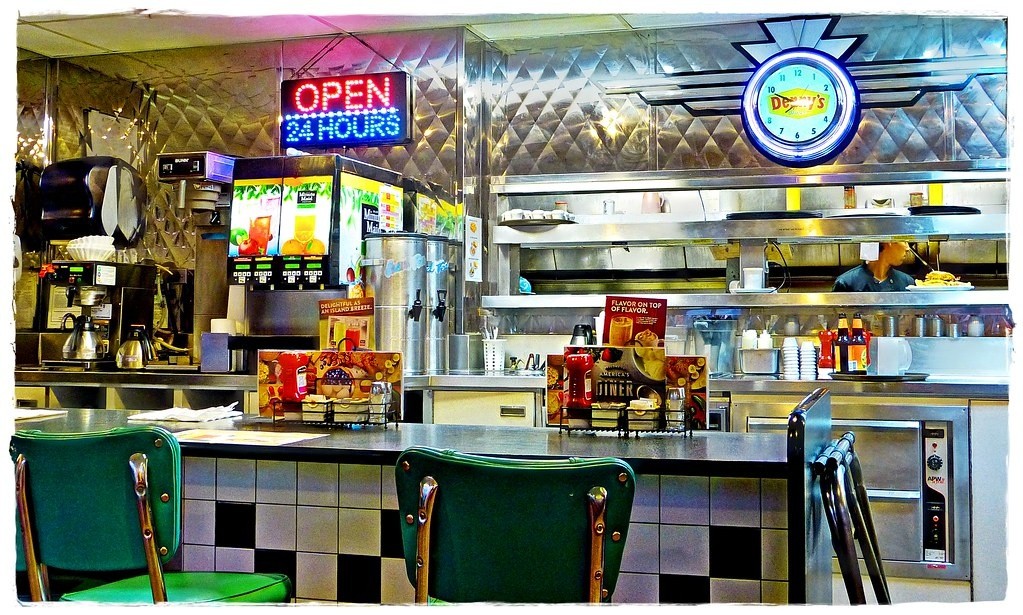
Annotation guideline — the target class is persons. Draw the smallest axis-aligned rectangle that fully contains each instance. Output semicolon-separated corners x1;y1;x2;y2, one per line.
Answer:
832;241;915;292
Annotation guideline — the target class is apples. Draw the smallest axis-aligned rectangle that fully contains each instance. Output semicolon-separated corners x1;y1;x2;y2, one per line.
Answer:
230;228;259;255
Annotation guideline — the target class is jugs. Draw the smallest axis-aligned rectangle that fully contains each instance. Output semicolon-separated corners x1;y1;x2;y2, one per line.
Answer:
878;337;912;375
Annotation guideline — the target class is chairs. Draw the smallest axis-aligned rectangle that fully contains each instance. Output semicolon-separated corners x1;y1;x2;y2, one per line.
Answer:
8;426;293;604
394;445;637;606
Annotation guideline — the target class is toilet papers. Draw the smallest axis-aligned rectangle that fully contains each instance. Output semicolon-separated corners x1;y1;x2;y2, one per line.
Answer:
210;318;237;372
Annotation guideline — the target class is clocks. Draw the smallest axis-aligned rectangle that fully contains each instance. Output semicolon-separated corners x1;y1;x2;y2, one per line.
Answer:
740;47;862;168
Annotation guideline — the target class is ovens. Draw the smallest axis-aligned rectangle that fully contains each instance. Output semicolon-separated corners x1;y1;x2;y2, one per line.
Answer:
746;416;951;563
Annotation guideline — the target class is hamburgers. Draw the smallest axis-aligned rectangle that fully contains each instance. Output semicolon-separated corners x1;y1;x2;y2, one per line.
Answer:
924;271;957;288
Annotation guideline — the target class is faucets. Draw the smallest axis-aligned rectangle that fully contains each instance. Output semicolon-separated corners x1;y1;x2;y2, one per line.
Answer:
509;357;526;370
433;289;447;323
408;287;423;323
60;313;76;330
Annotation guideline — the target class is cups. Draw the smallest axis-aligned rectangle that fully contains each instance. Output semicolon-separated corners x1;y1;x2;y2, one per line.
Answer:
609;315;633;346
945;316;984;337
503;208;575;220
786;183;944;210
782;337;816;374
743;268;762;289
882;316;943;337
604;192;666;213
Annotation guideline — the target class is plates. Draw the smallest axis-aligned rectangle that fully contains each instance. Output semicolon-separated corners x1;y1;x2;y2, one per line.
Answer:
905;286;974;290
734;289;773;293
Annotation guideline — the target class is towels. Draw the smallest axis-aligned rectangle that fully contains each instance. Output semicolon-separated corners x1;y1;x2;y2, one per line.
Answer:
14;407;68;425
126;400;244;422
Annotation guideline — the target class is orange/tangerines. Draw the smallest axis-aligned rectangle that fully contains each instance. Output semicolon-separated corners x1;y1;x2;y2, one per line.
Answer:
282;231;326;256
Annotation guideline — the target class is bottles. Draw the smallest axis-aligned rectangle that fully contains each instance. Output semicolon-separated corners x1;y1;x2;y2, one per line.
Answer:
742;329;773;349
784;318;800;335
566;354;594;407
844;184;856;209
818;313;871;380
278;353;392;423
157;350;190;365
554;201;568;211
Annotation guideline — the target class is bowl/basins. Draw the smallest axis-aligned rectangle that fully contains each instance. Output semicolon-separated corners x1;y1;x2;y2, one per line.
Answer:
66;236;116;261
548;409;560;420
631;348;653;380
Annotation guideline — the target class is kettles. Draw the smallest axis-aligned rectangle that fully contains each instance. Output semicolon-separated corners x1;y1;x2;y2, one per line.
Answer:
571;325;592;345
61;314;153;368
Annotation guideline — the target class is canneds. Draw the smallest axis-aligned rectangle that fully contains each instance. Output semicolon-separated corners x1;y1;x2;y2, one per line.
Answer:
909;192;923;207
844;185;855;208
554;201;568;212
